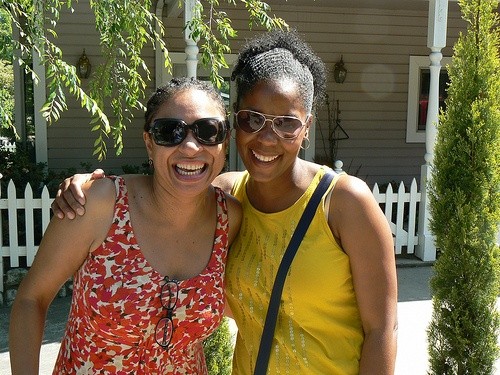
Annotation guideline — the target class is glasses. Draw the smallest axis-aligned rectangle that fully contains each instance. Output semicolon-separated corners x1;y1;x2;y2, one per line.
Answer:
147;118;229;147
234;109;311;139
154;276;181;349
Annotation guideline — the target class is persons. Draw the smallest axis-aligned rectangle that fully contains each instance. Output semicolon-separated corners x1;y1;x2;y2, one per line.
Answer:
51;27;398;375
8;79;243;375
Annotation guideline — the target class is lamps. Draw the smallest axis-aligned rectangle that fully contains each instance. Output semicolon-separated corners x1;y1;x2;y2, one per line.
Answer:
76;48;91;79
333;56;347;84
